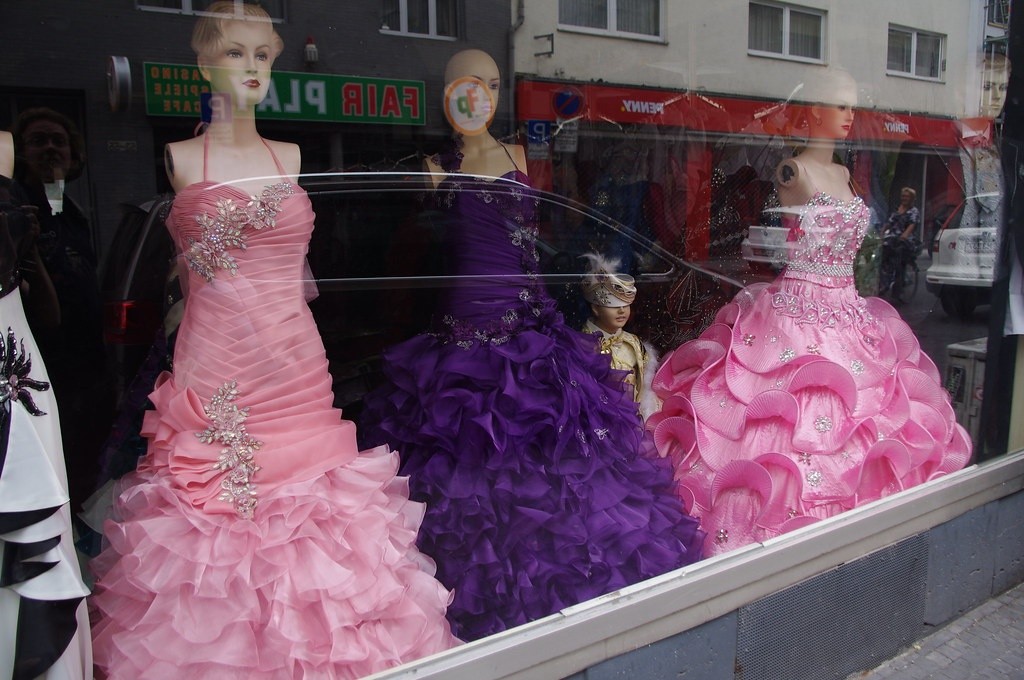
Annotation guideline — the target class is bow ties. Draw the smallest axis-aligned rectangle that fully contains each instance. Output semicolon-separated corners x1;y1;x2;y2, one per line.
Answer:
601;339;624;350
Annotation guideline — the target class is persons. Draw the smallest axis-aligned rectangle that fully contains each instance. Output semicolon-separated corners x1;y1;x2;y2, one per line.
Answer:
882;50;1016;465
1;130;93;680
365;46;706;642
639;66;973;558
1;108;107;557
574;254;659;422
91;3;471;680
881;185;922;300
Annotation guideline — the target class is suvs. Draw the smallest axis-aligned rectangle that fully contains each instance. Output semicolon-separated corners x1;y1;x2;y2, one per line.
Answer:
76;171;758;468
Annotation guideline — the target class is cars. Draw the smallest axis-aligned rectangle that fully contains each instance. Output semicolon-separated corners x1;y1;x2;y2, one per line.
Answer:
924;191;1002;319
741;205;859;277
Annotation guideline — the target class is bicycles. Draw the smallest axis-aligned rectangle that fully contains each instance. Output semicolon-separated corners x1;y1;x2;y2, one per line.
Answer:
855;228;919;303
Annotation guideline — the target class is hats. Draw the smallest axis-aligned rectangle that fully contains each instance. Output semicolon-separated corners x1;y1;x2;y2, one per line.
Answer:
577;253;637;307
